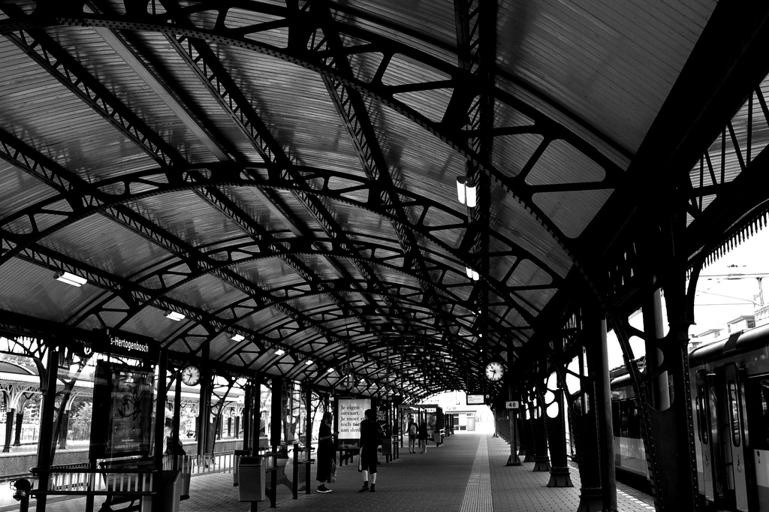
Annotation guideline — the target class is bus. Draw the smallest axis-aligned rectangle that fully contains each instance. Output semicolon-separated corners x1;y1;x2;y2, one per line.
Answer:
564;314;769;511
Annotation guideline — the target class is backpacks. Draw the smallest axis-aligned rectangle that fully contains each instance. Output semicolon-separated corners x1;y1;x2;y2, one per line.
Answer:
410;424;416;435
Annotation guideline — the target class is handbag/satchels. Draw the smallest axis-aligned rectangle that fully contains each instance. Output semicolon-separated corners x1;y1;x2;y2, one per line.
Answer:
330;459;336;473
358;448;362;472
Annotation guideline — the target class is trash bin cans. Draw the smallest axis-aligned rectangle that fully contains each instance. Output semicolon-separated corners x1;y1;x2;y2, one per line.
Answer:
240;458;265;511
434;432;441;447
204;454;211;467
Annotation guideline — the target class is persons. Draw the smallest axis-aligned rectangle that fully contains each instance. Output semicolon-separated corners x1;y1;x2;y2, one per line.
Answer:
167;434;191;499
418;421;427;453
313;411;339;493
406;418;418;453
356;408;386;491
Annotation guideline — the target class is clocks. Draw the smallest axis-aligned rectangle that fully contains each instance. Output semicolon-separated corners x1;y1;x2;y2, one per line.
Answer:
182;366;201;386
484;360;504;382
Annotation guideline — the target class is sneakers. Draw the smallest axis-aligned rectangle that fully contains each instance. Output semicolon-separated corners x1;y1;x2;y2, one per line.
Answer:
316;484;333;493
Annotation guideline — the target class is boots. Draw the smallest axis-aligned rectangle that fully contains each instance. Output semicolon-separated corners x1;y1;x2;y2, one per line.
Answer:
358;481;376;493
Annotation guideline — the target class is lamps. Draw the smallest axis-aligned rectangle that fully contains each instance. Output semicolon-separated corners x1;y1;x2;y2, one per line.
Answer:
53;270;88;287
305;357;313;365
163;309;185;322
273;347;286;356
465;262;480;282
229;331;245;343
455;161;477;208
